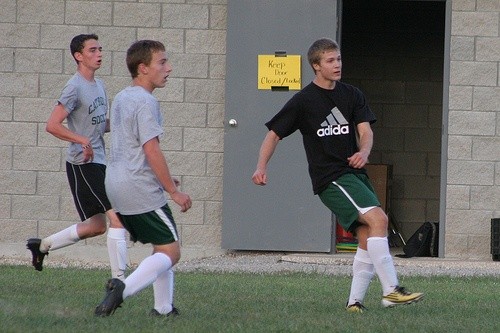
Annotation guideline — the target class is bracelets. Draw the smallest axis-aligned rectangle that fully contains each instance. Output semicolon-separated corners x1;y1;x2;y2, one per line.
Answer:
82;140;92;150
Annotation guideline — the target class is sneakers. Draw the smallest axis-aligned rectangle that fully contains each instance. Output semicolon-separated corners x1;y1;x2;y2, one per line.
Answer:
149;306;182;318
26;238;48;272
382;287;425;308
94;278;125;317
345;301;369;312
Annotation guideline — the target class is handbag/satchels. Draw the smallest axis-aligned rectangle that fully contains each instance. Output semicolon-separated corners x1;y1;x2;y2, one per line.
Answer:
394;223;439;256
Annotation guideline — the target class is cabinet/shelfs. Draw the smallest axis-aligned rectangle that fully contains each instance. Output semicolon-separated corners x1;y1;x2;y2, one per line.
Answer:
364;163;393;219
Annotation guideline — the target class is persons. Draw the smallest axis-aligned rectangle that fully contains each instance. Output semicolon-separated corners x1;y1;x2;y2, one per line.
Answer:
95;40;192;316
252;39;425;311
26;34;126;282
